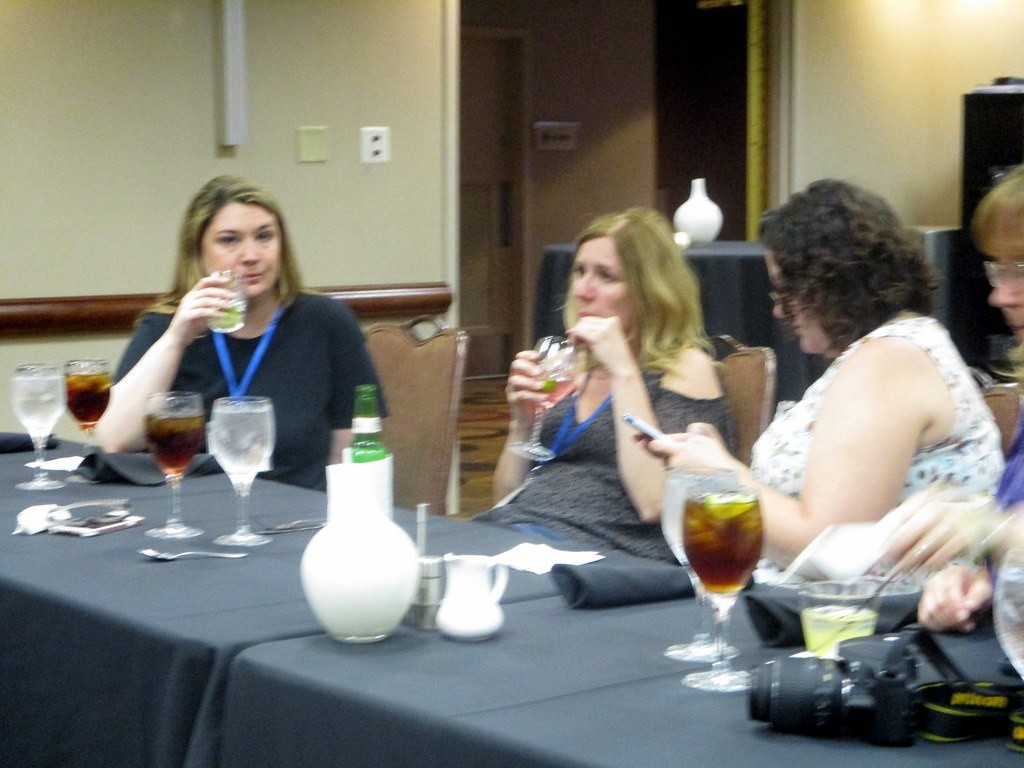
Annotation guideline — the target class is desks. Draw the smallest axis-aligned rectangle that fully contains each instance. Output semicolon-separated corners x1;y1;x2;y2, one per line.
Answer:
0;450;1024;768
545;240;834;401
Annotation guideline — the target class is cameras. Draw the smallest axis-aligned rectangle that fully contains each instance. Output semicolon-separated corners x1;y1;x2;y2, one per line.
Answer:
749;635;921;748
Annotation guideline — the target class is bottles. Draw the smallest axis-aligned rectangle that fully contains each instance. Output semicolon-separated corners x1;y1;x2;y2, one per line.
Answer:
346;382;386;464
672;177;724;248
301;489;423;643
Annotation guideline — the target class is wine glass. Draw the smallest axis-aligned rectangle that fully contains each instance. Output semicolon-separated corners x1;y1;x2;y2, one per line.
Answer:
9;360;66;492
681;480;764;694
210;396;277;548
506;335;589;464
139;390;206;541
64;356;111;484
662;465;743;663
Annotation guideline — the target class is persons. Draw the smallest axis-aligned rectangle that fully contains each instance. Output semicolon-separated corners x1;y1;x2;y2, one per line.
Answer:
634;179;1008;591
468;203;733;568
88;172;390;494
869;164;1024;636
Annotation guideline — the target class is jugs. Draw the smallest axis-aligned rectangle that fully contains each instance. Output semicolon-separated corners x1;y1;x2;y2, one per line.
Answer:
434;551;510;643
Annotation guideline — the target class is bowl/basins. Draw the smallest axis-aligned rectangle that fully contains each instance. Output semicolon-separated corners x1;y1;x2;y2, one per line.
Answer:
796;575;924;634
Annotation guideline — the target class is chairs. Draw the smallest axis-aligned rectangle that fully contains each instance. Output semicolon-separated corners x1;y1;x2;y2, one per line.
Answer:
325;315;467;515
712;335;775;468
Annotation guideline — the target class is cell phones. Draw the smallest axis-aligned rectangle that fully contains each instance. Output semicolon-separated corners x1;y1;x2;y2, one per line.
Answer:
624;413;667;441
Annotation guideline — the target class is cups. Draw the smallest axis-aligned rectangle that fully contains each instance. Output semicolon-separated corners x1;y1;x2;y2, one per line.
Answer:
991;543;1024;684
797;580;882;663
206;269;248;334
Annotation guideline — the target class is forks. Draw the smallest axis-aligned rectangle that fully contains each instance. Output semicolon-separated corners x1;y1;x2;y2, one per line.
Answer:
138;547;248;560
